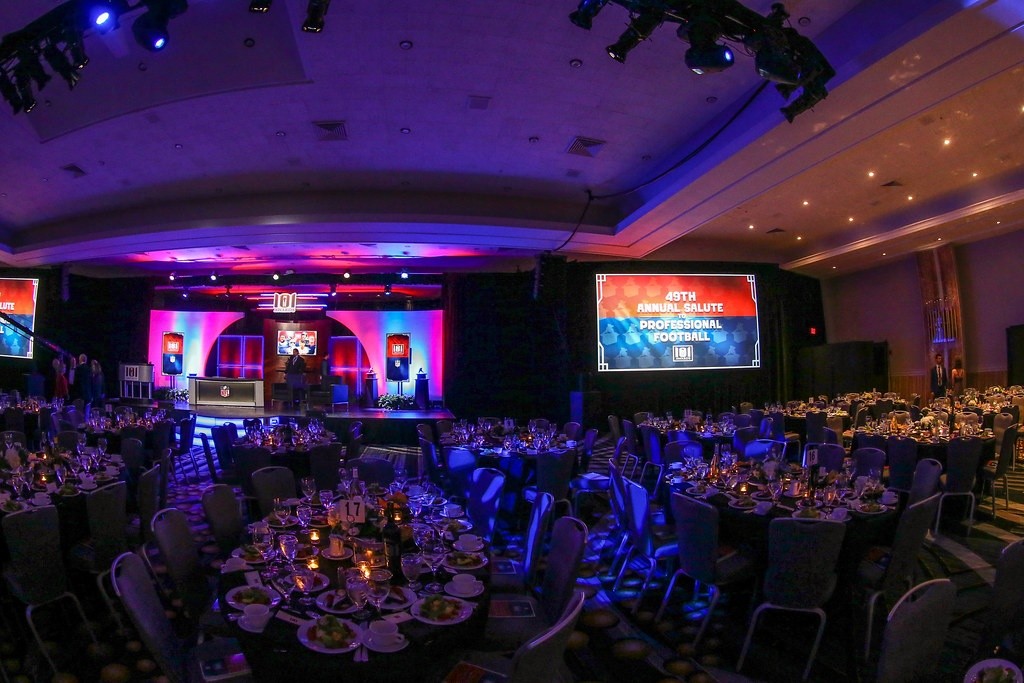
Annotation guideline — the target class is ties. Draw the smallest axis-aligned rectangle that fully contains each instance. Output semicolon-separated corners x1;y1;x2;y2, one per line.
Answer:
938;367;941;387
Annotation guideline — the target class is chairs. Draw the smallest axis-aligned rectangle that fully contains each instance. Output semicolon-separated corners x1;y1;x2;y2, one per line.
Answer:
0;375;1024;683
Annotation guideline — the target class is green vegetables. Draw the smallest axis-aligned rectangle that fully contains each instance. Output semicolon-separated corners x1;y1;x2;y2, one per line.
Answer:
5;501;23;511
234;590;271;604
419;595;453;619
447;551;468;564
238;543;259;558
319;615;349;648
61;486;78;494
798;510;817;518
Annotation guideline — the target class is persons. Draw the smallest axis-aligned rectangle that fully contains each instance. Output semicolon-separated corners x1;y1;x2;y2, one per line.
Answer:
952;359;966;395
279;334;288;347
301;333;307;343
46;354;103;403
306;337;315;354
118;449;147;485
286;339;299;354
320;352;330;397
930;354;948;398
298;340;310;354
285;348;305;406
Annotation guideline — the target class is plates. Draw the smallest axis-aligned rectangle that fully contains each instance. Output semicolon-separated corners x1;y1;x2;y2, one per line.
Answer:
239;551;276;564
439;511;464;518
285;570;330;592
367;586;418;610
238;615;274;633
456;520;473;532
281;544;319;560
316;590;363;613
321;547;353;559
410;595;473;625
361;629;409;652
225;584;281;610
442;552;488;570
297;617;363;653
366;487;389;495
452;540;485;552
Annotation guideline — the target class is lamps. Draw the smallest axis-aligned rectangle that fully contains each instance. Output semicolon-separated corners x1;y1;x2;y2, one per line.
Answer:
272;271;281;280
567;0;664;62
675;16;735;76
0;34;90;115
90;0;190;52
331;269;351;296
248;0;329;33
385;267;410;295
774;78;829;123
211;271;231;298
169;271;191;299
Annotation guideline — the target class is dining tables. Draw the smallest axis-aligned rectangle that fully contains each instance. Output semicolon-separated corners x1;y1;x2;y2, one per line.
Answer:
248;426;337;475
637;421;734;458
77;423;160;454
439;429;579;477
0;403;51;438
849;418;989;469
665;464;899;620
0;445;129;542
200;481;492;683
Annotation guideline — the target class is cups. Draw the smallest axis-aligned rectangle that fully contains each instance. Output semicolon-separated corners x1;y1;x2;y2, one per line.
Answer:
368;621;406;647
458;534;482;552
444;504;461;516
242;604;269;628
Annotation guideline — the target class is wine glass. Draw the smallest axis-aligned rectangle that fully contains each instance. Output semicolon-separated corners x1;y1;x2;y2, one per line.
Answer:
0;390;65;413
681;442;881;519
89;404;166;431
453;417;557;454
645;410;737;436
0;431;108;512
253;467;450;620
245;417;325;450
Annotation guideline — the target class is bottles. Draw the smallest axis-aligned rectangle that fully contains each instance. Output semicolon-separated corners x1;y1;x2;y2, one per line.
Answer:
349;469;363;500
381;502;401;573
